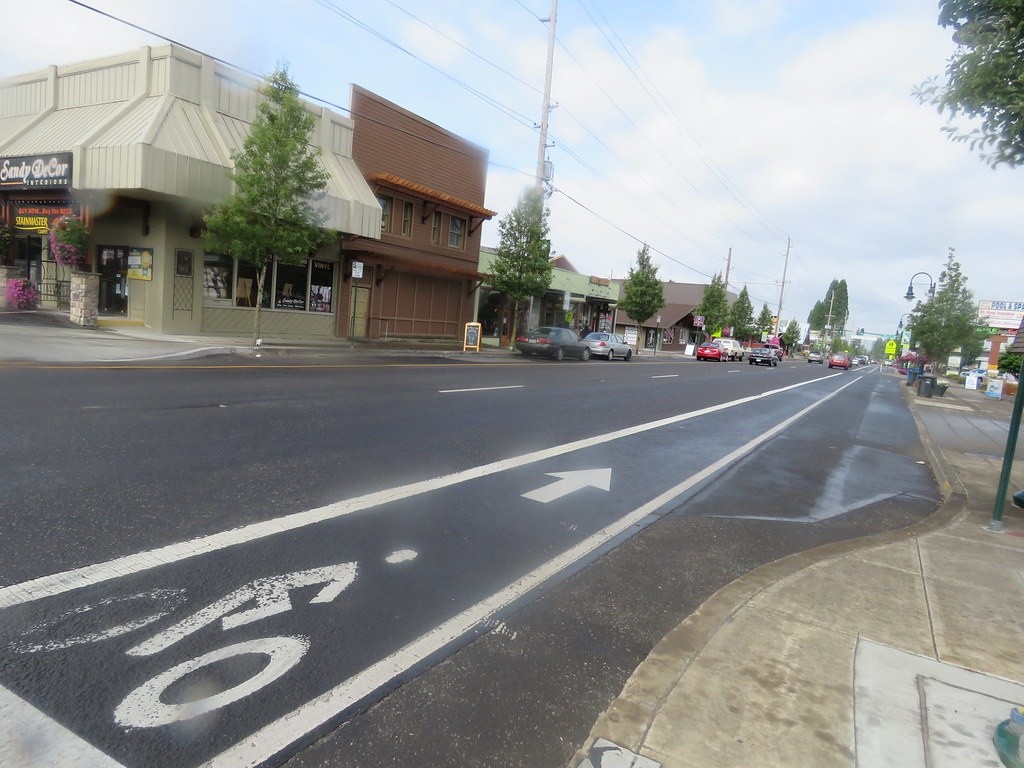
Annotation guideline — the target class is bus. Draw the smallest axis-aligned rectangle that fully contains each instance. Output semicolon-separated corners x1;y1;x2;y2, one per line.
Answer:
800;344;832;356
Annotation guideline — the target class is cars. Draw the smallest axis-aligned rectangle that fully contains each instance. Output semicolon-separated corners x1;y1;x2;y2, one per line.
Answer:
695;342;729;362
515;326;590;360
582;332;632;361
959;369;988;383
762;344;783;361
748;348;780;367
883;359;893;366
851;355;879;364
828;353;848;370
807;351;824;364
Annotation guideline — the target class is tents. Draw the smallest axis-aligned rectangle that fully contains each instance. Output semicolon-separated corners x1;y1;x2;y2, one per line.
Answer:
897;354;928;373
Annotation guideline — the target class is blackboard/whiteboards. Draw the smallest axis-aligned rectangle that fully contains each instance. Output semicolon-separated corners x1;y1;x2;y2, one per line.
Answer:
464;322;481;348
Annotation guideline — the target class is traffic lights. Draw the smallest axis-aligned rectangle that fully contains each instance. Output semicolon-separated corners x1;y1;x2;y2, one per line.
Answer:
861;329;864;335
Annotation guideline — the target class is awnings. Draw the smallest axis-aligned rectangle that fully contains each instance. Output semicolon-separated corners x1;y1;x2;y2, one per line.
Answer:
344;248;486;298
369;173;499;235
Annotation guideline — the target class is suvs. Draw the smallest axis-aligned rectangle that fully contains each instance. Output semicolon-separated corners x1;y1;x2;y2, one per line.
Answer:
713;339;745;362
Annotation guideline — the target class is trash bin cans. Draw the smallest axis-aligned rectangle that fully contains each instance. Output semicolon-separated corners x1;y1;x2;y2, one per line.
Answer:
917;375;936;398
906;368;923;384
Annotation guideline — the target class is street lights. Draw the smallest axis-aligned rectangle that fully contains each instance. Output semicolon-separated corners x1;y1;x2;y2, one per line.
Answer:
903;271;937;376
900;312;915;334
